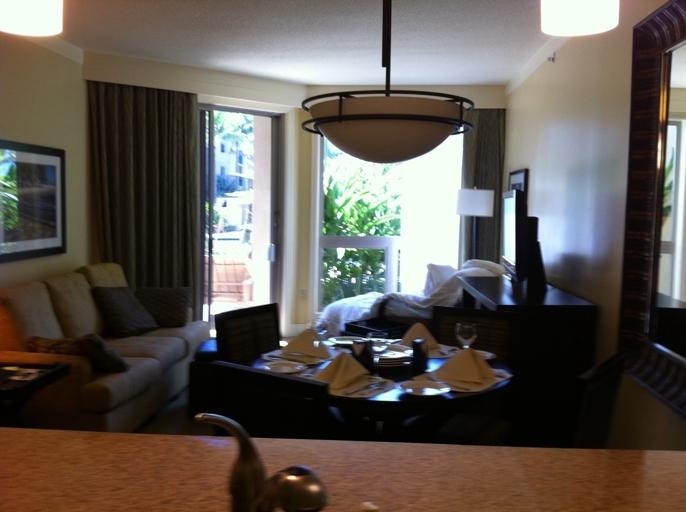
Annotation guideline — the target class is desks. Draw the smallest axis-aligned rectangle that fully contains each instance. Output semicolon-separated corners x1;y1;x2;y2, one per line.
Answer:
456;275;597;447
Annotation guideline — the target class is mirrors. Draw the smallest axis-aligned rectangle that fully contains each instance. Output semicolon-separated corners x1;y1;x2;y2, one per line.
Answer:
618;0;686;418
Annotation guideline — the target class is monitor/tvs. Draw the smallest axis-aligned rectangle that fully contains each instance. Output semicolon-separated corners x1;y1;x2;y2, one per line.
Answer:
499;188;546;289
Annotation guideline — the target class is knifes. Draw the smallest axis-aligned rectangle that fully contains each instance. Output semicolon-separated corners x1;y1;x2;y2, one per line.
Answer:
267;353;308;364
343;380;387;396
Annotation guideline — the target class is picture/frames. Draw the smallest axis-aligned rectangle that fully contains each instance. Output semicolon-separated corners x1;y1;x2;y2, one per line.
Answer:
508;168;529;213
0;139;67;262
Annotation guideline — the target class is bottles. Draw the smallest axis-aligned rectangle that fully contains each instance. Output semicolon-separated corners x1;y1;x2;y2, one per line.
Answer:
411;338;428;372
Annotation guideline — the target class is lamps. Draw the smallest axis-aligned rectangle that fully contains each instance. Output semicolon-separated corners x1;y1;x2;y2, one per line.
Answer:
299;0;475;164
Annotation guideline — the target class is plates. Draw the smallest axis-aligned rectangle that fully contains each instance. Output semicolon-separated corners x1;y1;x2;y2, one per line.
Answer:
330;336;364;345
370;344;413;367
399;379;453;396
263;360;308;374
451;349;498;360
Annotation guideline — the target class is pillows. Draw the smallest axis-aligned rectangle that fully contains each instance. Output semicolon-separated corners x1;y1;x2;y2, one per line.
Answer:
92;284;161;340
424;264;454;299
23;333;131;374
135;286;192;327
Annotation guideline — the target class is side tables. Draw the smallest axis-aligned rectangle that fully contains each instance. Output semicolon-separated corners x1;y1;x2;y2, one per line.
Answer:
0;362;71;427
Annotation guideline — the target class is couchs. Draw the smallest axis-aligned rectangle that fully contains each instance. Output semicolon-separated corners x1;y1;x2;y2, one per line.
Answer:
0;262;210;434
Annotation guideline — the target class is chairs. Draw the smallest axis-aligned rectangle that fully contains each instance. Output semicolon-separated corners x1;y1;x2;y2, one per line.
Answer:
210;361;332;441
405;389;517;445
315;256;506;346
213;303;281;365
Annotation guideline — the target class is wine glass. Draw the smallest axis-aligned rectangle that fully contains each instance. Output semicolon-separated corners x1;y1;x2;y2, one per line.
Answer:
455;321;478;354
